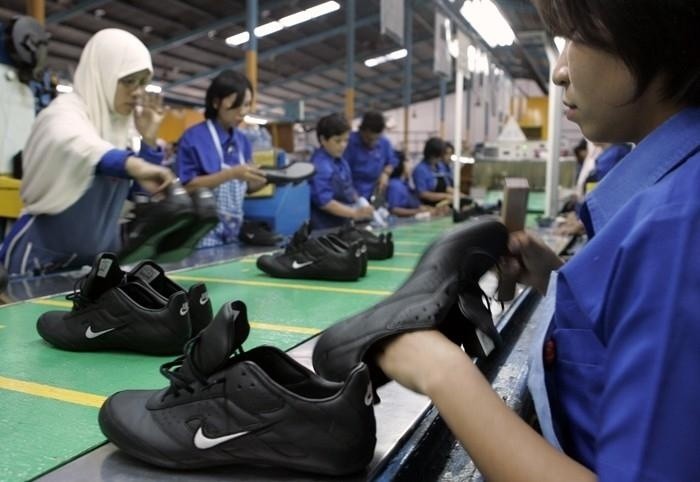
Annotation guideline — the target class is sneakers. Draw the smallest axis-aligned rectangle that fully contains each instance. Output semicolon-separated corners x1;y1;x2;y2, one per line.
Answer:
117;179;219;266
97;299;377;482
36;251;191;357
257;223;393;282
129;260;213;337
312;222;508;406
257;161;317;187
449;197;502;223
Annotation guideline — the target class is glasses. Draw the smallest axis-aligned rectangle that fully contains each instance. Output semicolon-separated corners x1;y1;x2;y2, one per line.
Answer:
119;76;152;87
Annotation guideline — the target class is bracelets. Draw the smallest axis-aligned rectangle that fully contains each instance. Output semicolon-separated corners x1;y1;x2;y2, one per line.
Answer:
383;171;391;177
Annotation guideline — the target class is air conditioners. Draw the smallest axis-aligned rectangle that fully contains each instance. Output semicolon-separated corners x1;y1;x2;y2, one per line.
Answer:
459;0;517;49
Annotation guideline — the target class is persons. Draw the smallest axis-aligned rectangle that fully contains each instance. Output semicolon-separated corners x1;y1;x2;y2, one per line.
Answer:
307;111;380;233
1;27;179;301
376;1;700;481
443;141;456;208
174;65;297;253
343;109;398;207
411;137;472;212
552;132;635;244
385;145;451;219
